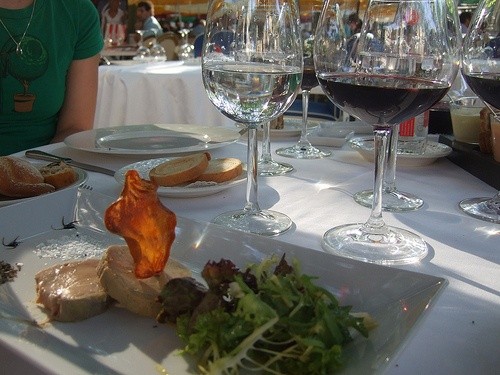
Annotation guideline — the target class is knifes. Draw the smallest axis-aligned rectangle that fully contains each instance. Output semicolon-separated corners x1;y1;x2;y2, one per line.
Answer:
25;150;115;178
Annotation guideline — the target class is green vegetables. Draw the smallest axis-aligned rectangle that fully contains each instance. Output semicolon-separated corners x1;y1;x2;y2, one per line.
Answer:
158;251;379;375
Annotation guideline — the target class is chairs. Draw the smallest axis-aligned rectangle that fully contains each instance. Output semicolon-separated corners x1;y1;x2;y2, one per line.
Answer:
128;13;380;121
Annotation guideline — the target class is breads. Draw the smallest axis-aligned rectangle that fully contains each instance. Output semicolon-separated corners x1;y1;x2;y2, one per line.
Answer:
149;152;243;185
270;115;285;130
0;155;74;198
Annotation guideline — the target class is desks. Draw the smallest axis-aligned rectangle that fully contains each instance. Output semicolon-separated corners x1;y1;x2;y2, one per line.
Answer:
103;48;138;57
0;141;499;375
92;55;241;129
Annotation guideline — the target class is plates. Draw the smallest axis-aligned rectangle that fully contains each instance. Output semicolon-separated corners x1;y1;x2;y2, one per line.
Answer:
0;157;88;206
308;120;372;147
350;135;450;169
114;157;250;197
65;123;240;154
0;188;450;375
255;119;319;136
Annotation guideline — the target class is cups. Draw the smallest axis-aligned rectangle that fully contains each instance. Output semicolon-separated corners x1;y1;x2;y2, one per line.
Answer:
451;96;500;166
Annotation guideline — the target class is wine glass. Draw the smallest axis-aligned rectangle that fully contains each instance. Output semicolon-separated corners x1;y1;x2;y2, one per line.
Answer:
313;0;457;267
458;0;500;224
273;3;348;160
201;0;303;237
352;1;452;212
236;3;303;176
133;28;194;62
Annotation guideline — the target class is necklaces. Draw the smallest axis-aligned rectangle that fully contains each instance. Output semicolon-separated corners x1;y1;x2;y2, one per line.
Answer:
0;0;36;55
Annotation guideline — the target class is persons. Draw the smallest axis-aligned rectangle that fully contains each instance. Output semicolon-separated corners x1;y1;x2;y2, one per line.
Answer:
346;12;364;38
97;0;165;48
459;12;472;34
0;0;105;158
